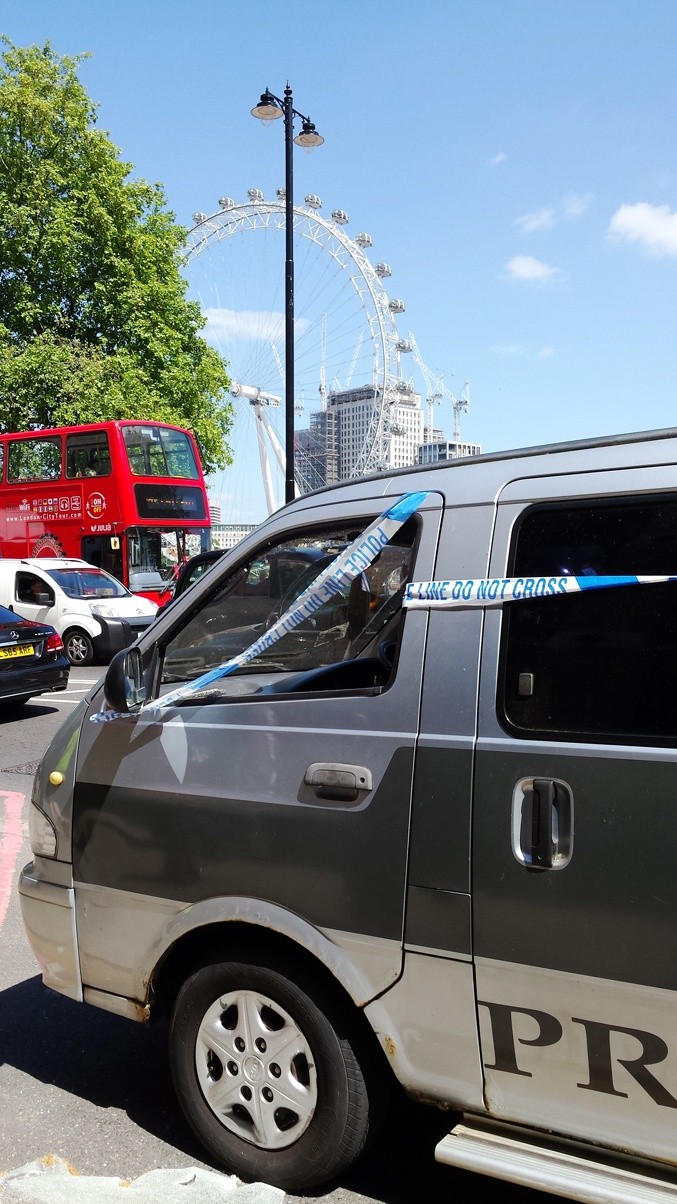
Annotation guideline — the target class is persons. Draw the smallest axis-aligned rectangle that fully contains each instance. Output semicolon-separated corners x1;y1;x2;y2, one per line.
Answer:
128;456;137;473
24;580;45;602
172;546;189;580
84;458;102;476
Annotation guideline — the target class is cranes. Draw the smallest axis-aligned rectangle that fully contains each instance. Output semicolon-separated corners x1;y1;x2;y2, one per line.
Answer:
405;330;471;442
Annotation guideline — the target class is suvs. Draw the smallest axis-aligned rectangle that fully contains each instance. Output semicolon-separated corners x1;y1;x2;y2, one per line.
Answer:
152;546;412;685
0;558;159;667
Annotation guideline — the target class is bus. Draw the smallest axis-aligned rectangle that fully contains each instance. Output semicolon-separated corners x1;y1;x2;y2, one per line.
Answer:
0;419;213;608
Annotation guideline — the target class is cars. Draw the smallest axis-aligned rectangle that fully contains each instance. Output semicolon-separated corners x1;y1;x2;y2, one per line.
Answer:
0;605;71;706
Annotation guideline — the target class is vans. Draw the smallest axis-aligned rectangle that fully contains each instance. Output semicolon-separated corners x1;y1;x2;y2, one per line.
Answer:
16;432;676;1204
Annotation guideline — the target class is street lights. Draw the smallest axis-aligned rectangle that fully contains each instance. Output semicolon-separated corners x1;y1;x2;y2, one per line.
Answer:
250;79;326;503
